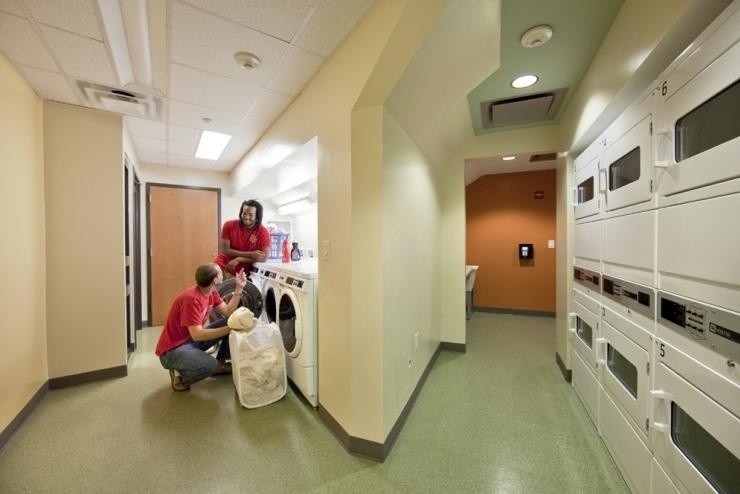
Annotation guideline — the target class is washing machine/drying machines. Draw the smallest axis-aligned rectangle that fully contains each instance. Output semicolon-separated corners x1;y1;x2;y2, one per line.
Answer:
209;258;318;409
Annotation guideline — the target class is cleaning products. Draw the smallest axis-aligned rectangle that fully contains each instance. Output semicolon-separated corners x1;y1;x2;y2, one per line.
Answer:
279;233;290;263
291;243;300;261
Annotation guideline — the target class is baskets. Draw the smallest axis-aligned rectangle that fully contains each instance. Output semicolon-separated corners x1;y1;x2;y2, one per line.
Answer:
267;232;290;259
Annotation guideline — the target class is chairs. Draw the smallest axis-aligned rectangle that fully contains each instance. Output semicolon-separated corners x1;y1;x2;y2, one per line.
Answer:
465;264;480;320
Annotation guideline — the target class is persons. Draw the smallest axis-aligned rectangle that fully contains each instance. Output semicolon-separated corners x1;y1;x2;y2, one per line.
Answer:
155;262;247;391
214;199;271;280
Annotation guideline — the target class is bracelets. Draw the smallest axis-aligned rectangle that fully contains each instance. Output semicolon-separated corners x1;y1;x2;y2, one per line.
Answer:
234;293;242;297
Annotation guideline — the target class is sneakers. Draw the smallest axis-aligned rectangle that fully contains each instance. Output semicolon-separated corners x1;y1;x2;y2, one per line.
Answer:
211;358;232;375
170;367;189;391
